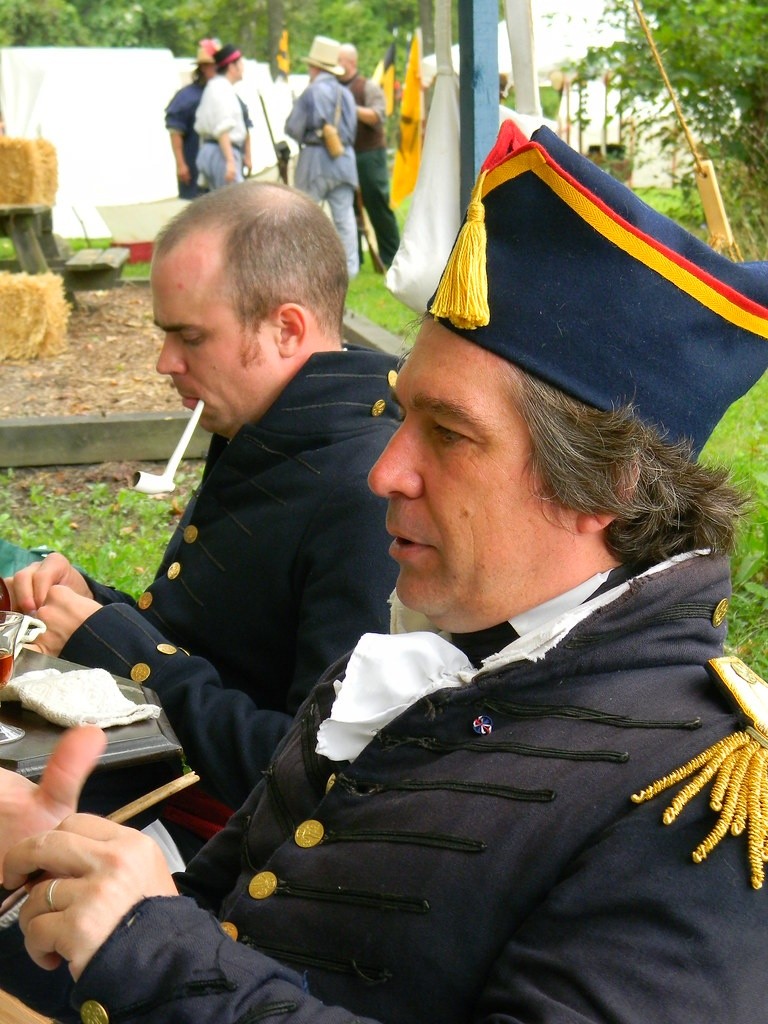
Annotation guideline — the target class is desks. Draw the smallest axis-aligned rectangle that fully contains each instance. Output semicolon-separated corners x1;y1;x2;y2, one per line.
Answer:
0;626;185;821
1;197;79;319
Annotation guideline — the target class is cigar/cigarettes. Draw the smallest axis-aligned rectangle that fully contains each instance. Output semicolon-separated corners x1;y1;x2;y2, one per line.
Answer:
132;400;208;495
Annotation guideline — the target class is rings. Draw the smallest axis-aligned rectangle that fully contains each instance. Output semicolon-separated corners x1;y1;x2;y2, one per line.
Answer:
44;878;58;912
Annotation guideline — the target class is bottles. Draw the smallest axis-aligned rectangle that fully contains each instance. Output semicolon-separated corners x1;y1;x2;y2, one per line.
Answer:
320;118;345;158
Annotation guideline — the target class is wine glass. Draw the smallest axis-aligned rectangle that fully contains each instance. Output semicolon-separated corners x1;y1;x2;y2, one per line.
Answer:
0;611;26;744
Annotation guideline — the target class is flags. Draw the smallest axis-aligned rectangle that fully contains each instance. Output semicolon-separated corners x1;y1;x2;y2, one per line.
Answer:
381;26;424;212
370;41;396;161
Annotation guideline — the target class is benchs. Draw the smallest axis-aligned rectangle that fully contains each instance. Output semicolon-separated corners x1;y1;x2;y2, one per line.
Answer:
50;240;131;293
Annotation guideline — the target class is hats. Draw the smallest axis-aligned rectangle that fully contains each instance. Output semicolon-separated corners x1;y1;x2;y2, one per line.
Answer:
426;117;768;465
212;43;244;73
192;39;223;64
301;34;345;75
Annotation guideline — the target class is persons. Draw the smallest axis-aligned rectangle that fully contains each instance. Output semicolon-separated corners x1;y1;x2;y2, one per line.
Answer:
334;43;400;271
284;36;359;279
193;43;248;192
165;37;254;201
4;180;407;865
0;118;767;1024
